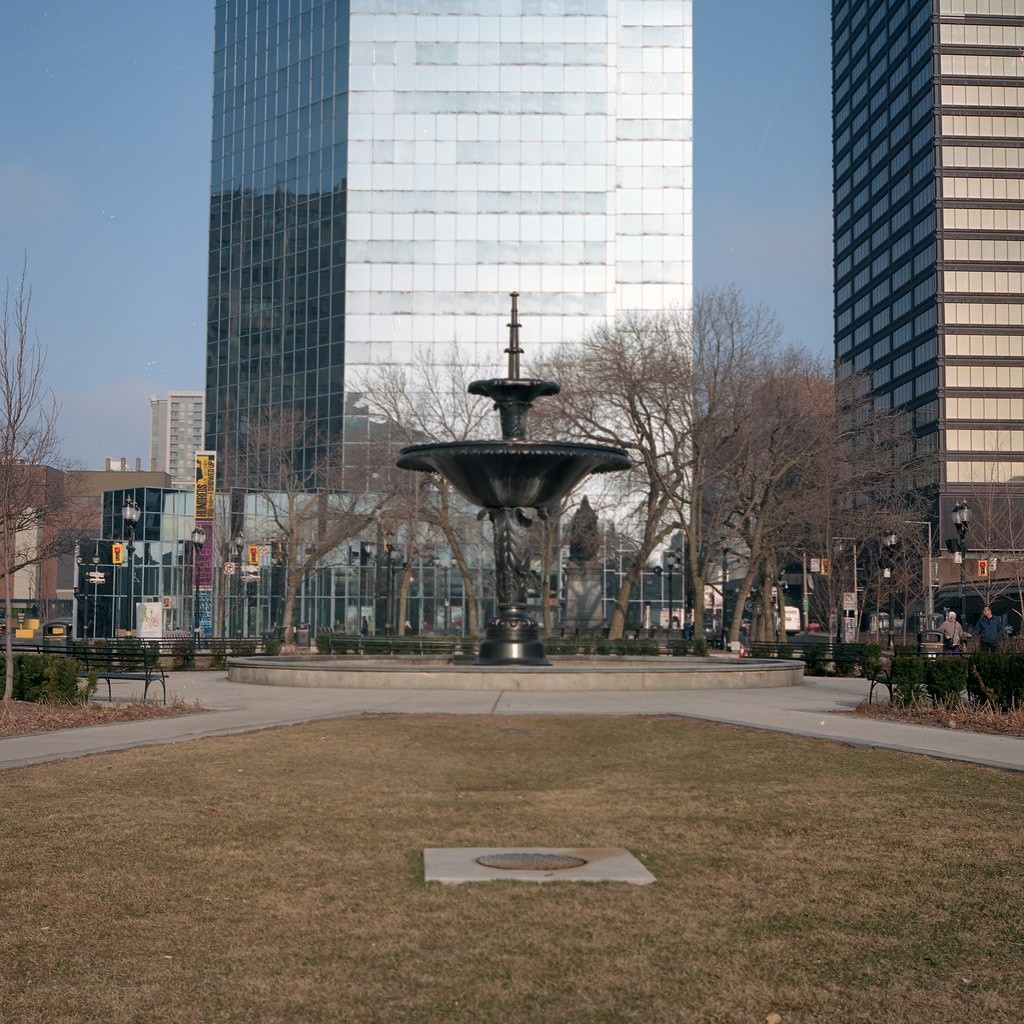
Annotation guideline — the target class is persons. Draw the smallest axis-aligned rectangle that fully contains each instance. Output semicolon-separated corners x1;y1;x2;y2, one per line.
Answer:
973;606;1004;652
1004;623;1013;636
684;617;822;642
936;611;973;656
282;615;463;646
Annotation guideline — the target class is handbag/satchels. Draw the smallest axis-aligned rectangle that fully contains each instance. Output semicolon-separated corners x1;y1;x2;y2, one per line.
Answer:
944;638;953;651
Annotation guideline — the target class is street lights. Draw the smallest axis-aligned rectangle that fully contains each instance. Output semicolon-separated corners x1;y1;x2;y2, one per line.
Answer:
668;548;681;629
364;544;398;634
235;530;245;641
191;527;205;650
883;528;897;650
76;556;99;640
952;496;972;634
434;556;457;637
122;494;141;635
719;537;730;650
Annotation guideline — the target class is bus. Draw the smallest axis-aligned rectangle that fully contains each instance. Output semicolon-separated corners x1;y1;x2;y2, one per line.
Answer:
773;606;800;636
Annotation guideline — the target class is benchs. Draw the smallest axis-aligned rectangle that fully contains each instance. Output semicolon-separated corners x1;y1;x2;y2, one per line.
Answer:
328;636;480;654
867;649;1024;708
741;640;869;677
0;644;170;705
105;633;270;672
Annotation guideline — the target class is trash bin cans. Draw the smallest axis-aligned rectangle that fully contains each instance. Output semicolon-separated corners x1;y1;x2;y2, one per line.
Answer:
296;622;311;647
43;622;73;659
917;630;946;658
